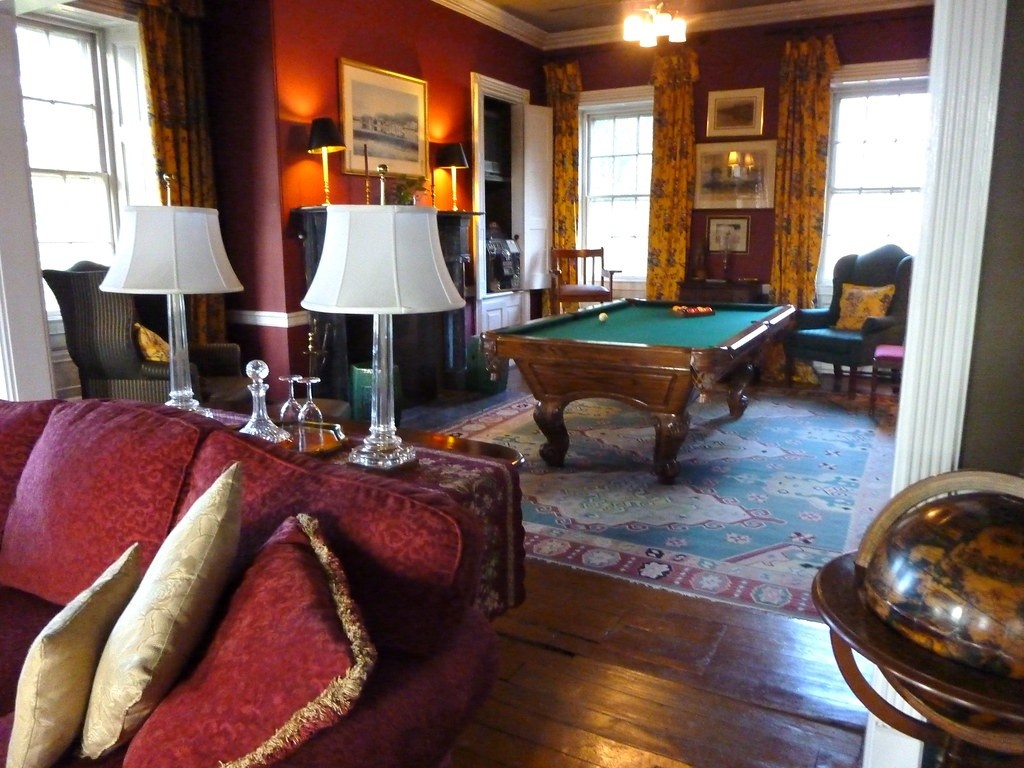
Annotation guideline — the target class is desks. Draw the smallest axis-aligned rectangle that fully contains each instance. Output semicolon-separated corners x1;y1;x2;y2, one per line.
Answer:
482;297;796;484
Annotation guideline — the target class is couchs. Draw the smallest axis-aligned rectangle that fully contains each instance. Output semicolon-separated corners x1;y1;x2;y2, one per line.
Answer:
0;399;494;768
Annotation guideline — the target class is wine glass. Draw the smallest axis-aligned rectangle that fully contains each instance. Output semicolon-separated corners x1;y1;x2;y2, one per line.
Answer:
297;377;323;423
278;375;302;423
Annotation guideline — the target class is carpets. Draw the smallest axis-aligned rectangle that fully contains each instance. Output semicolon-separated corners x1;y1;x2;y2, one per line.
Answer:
425;380;897;622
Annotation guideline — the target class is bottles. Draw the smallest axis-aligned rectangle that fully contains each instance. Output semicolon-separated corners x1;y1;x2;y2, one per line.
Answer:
238;359;294;449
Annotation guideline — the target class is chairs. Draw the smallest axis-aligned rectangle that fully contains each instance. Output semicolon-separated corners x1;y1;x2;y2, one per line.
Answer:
549;247;622;314
794;243;914;400
43;260;263;413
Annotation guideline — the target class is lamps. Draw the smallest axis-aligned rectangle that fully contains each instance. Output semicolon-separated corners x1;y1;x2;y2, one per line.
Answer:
300;164;466;467
433;143;469;210
727;151;755;171
100;177;244;419
621;2;687;49
307;116;347;206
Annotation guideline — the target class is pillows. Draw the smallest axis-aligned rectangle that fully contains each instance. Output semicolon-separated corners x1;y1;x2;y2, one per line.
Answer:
0;399;377;767
836;283;895;333
132;322;171;363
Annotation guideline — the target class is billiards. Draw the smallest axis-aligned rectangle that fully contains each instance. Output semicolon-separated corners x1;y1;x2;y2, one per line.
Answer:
672;305;713;313
599;312;608;322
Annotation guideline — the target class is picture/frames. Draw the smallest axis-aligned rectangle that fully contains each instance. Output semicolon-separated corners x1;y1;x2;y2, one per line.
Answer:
705;214;750;255
335;54;429;180
693;139;777;210
706;87;764;138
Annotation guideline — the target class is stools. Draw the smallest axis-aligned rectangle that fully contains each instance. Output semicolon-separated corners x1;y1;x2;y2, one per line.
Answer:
869;344;904;412
